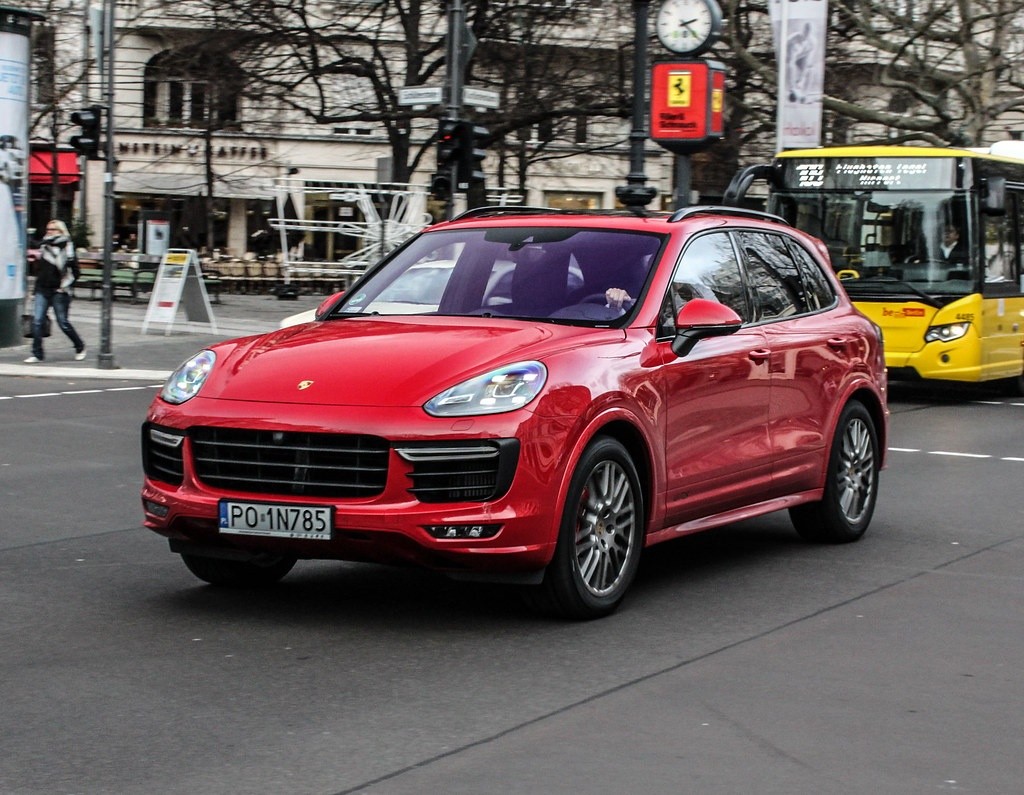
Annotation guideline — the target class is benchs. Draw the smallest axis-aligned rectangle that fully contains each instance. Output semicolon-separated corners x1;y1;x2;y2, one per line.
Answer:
70;254;222;305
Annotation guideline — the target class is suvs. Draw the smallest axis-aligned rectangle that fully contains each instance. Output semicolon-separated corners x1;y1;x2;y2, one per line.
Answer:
140;205;886;628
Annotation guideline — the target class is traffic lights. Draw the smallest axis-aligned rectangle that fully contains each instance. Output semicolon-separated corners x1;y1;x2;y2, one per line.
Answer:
457;120;490;183
436;116;461;166
70;105;102;160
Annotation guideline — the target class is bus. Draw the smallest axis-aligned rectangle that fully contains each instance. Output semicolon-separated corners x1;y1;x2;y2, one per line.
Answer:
722;140;1024;400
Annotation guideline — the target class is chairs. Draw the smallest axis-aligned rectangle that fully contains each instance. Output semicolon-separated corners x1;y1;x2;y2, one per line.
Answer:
511;243;585;314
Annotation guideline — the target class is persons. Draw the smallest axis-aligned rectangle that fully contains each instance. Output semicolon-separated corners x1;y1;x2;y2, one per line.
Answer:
930;221;969;265
603;251;688;327
23;220;87;363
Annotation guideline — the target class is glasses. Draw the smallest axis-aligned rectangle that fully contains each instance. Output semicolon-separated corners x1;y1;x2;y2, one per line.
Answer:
46;227;60;232
941;229;957;235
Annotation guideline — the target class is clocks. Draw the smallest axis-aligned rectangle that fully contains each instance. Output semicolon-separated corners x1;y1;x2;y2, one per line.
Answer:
656;0;722;59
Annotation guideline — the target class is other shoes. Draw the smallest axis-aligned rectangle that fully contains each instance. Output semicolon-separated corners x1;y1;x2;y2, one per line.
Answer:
75;344;86;360
24;357;43;362
